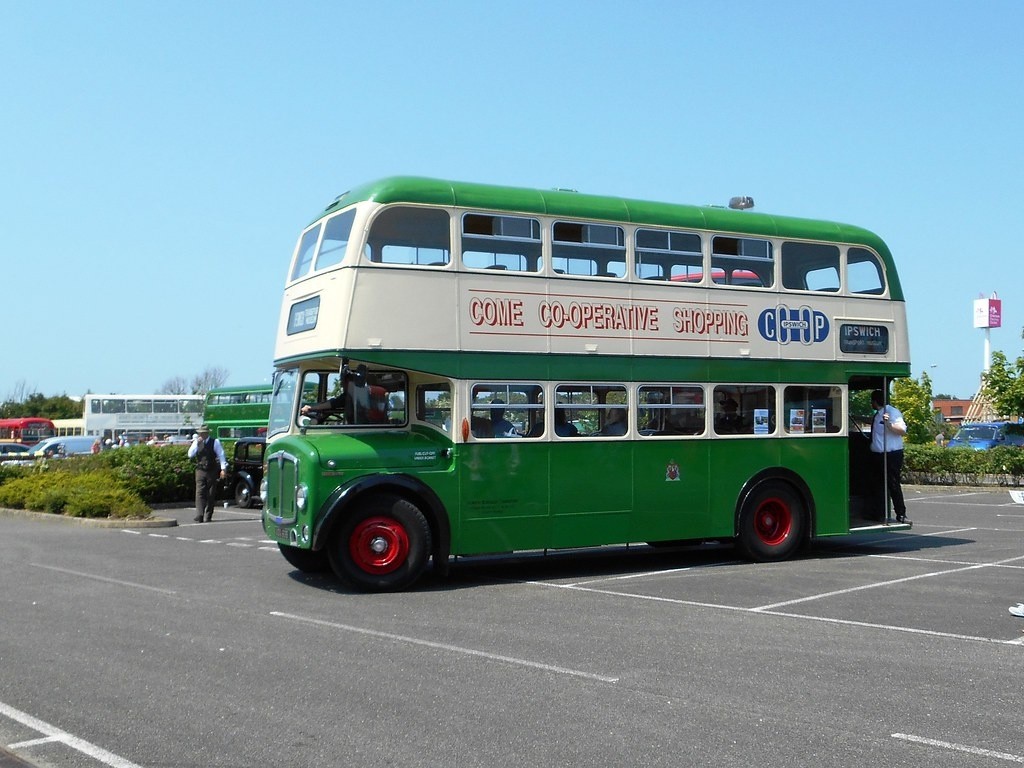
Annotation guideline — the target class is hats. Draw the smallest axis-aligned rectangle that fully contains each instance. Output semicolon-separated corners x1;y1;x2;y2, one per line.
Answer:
195;424;214;433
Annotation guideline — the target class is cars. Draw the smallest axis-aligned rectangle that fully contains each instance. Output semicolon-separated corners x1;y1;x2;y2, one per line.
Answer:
0;442;32;459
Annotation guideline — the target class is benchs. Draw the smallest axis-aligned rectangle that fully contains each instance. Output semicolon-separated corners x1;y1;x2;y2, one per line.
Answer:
430;262;840;293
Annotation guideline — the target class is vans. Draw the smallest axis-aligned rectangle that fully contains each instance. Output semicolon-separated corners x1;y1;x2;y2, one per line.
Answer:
28;435;104;459
226;436;267;511
945;422;1024;452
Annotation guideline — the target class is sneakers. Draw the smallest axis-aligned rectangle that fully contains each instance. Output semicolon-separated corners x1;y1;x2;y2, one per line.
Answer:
1008;602;1024;617
896;515;913;526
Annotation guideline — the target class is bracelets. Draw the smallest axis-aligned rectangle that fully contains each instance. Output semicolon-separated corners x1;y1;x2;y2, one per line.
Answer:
887;421;892;428
221;469;227;472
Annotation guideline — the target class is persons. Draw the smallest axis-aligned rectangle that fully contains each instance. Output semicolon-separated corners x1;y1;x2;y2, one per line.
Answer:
43;443;67;459
91;430;171;455
863;390;913;525
300;365;390;424
445;398;746;438
935;430;947;447
187;424;227;522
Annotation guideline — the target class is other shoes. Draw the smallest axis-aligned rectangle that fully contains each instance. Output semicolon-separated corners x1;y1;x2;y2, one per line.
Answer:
204;517;212;523
194;515;204;523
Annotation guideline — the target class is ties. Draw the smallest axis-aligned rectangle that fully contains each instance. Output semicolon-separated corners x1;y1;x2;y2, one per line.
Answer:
203;440;207;448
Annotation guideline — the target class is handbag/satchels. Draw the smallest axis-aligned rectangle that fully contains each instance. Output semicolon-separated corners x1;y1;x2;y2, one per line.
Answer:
210;477;226;501
495;427;523;438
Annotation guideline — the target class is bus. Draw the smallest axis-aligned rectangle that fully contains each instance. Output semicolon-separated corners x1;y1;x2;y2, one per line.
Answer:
262;176;912;594
80;393;255;443
0;418;56;446
201;381;318;445
50;419;85;437
